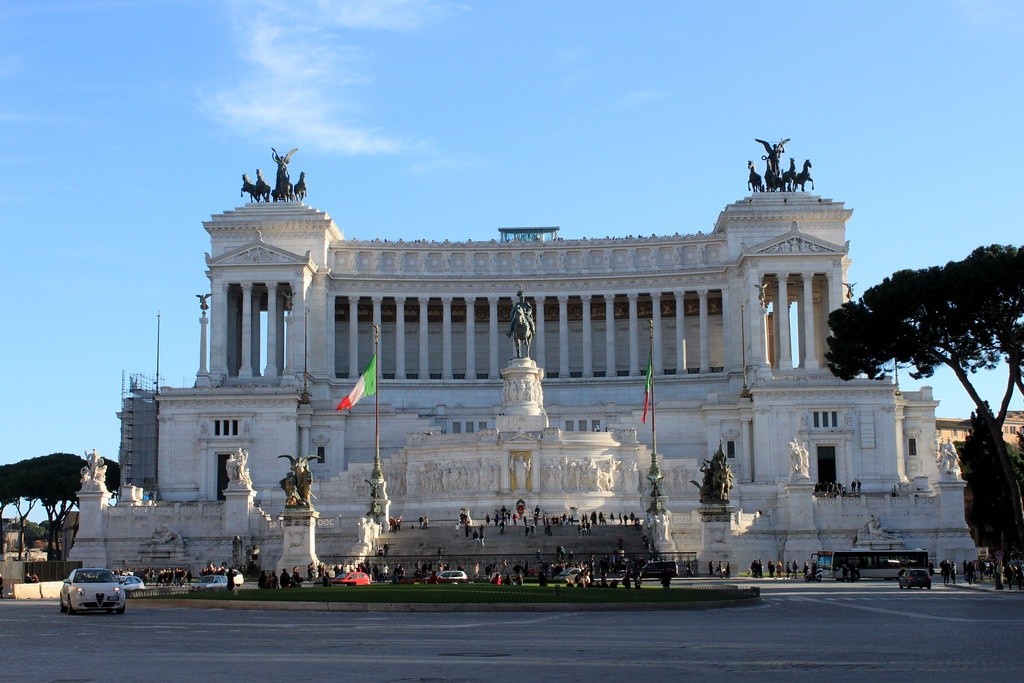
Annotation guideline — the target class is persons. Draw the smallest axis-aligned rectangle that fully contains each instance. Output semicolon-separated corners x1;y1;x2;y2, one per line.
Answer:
506;288;536;339
24;572;39;583
700;451;734;500
815;480;861;497
110;504;731;590
226;447;251;482
869;515;899;539
81;449;107;484
746;557;1024;590
284;457;312;505
787;436;809;477
350;454;691;496
768;144;779;172
277;156;287;171
938;437;962;470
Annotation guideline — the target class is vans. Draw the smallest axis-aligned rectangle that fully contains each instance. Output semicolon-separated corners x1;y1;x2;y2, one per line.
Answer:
641;559;679;578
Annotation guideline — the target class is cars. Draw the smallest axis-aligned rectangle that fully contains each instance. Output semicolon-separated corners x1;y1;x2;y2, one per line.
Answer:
193;574;228;588
554;568;582;584
117;575;146;591
331;571;371;587
422;570;467;580
899;569;932;590
223;568;245;587
59;568;126;615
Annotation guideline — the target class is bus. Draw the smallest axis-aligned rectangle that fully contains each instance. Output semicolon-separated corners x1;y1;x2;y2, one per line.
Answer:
811;547;930;581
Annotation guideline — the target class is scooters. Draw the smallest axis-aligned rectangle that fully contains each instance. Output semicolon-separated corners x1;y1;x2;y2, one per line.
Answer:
804;567;823;582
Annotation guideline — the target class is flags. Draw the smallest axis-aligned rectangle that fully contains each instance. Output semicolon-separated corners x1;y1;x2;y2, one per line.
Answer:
336;352;377;413
642;352;652;424
